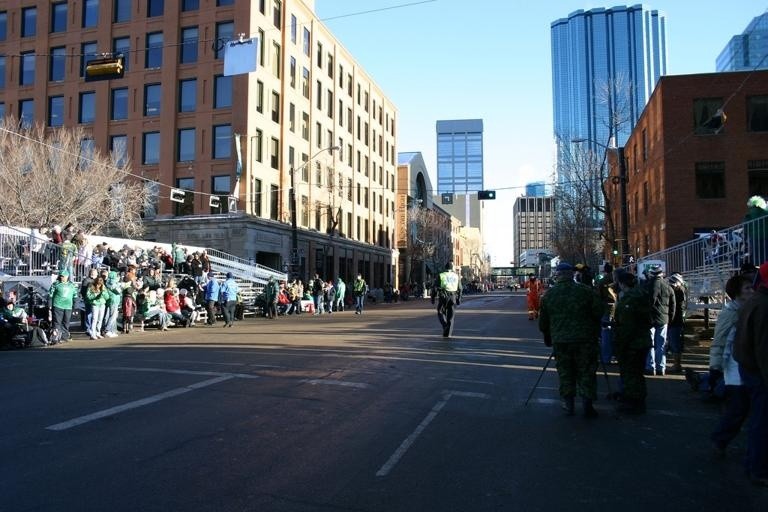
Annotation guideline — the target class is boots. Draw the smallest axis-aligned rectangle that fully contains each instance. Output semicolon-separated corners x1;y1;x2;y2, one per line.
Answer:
562;396;576;413
582;397;599;418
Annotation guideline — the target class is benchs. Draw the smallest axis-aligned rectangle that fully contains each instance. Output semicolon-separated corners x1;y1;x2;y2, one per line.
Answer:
0;258;264;333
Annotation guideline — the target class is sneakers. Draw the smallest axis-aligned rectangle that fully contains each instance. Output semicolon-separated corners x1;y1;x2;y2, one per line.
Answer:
614;400;649;412
88;330;121;341
312;306;364;315
648;353;683;377
161;314;247;332
261;309;302;320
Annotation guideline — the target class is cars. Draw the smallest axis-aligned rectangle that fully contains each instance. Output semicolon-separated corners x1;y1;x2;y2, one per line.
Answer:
547;283;554;289
513;284;521;289
497;284;506;290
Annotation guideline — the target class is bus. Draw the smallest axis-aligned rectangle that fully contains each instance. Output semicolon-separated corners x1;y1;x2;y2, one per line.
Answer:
540;277;552;289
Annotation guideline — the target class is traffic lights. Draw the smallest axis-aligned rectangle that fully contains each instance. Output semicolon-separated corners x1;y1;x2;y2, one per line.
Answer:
477;189;495;201
209;195;219;208
170;189;185;203
283;265;289;274
612;248;619;258
622;254;635;265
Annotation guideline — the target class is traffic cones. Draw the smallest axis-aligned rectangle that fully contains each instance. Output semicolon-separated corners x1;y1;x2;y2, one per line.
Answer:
308;304;313;313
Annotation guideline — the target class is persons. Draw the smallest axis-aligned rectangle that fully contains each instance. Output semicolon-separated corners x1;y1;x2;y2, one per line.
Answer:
462;281;495;294
255;273;369;316
524;259;688;417
707;262;768;485
383;281;423;304
431;262;463;337
0;222;244;345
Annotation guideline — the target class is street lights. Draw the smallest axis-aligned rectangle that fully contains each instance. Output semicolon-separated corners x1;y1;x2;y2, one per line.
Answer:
286;145;343;279
568;138;630;271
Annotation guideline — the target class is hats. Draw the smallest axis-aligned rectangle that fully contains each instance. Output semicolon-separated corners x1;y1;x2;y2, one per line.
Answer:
227;271;233;278
649;265;663;273
669;275;679;283
207;272;214;278
556;262;573;269
60;271;70;277
760;260;768;288
108;271;118;279
